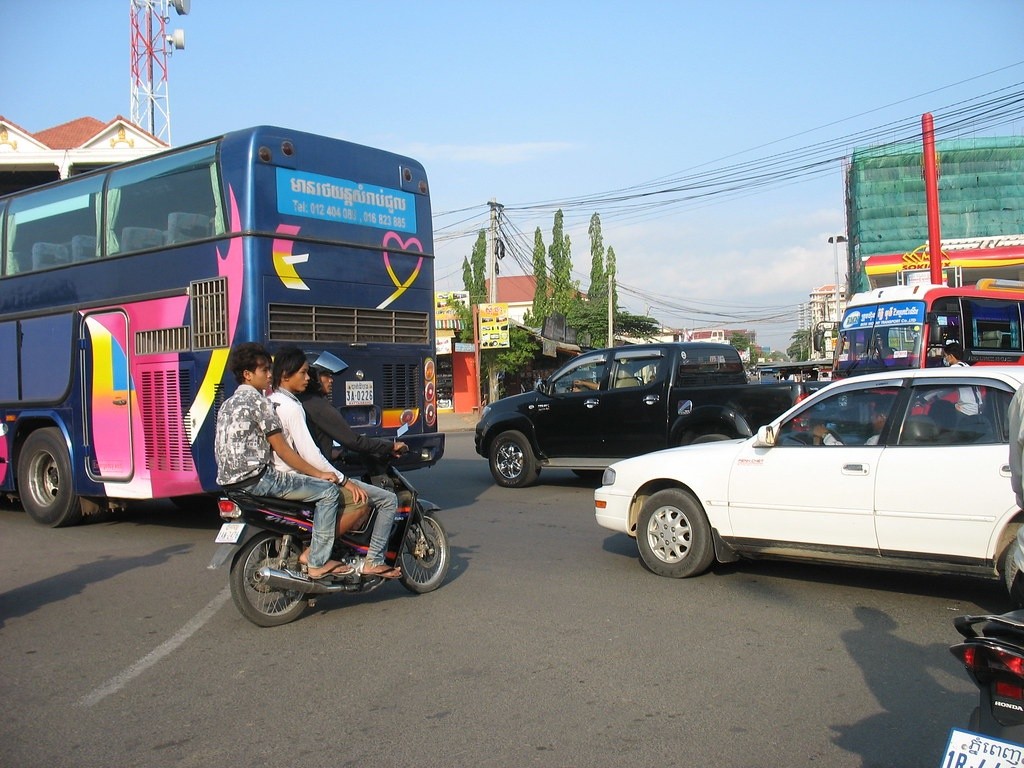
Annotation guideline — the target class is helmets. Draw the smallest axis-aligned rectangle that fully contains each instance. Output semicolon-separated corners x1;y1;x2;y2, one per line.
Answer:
304;350;349;396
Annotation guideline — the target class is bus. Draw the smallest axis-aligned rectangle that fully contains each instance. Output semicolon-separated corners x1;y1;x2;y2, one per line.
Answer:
0;125;445;528
813;277;1024;434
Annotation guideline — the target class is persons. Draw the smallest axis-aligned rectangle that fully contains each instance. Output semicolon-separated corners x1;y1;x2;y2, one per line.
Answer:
918;343;982;415
806;394;893;447
1007;384;1024;614
213;341;408;579
570;379;600;392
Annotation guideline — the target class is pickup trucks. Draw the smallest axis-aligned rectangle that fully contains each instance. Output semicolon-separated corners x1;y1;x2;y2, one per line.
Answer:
474;342;856;489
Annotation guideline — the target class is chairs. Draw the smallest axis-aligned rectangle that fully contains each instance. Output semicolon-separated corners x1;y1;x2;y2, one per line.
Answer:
901;400;991;446
978;329;1003;348
31;213;216;271
615;364;641;388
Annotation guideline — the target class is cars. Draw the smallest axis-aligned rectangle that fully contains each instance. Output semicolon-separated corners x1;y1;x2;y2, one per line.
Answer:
593;365;1024;610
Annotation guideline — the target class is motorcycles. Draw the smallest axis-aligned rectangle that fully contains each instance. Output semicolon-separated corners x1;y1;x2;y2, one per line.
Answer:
205;423;450;628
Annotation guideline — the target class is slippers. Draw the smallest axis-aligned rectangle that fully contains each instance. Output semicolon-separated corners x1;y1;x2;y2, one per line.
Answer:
309;561;356;580
361;567;403;579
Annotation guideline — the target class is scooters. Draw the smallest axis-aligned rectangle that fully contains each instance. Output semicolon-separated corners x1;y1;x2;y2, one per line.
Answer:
938;611;1024;768
